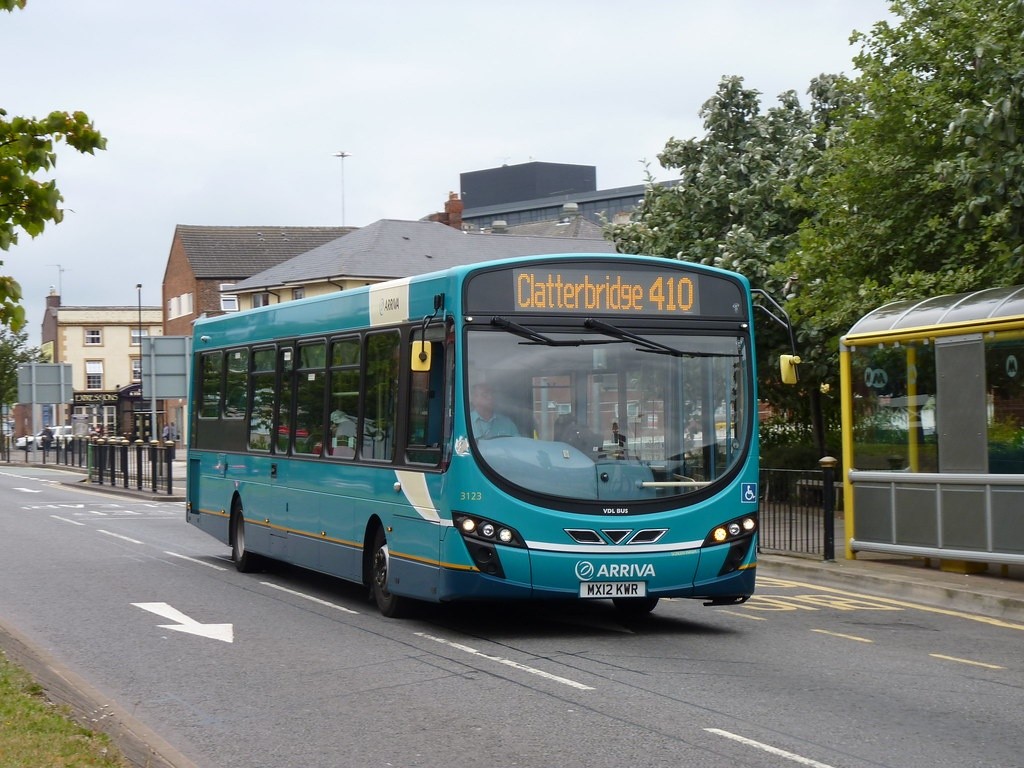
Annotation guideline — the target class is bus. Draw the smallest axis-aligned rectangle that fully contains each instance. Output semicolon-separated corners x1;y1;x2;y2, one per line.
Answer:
185;251;761;628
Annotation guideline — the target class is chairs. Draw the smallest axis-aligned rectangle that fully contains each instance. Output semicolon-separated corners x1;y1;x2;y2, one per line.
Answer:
555;414;603;461
250;435;358;459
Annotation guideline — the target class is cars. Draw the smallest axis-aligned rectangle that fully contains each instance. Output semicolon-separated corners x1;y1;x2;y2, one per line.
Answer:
15;425;74;452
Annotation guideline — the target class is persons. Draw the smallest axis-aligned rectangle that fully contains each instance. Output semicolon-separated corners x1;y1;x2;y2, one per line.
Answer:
469;384;523;441
312;410;353;458
41;424;54;457
170;422;180;444
162;423;169;441
